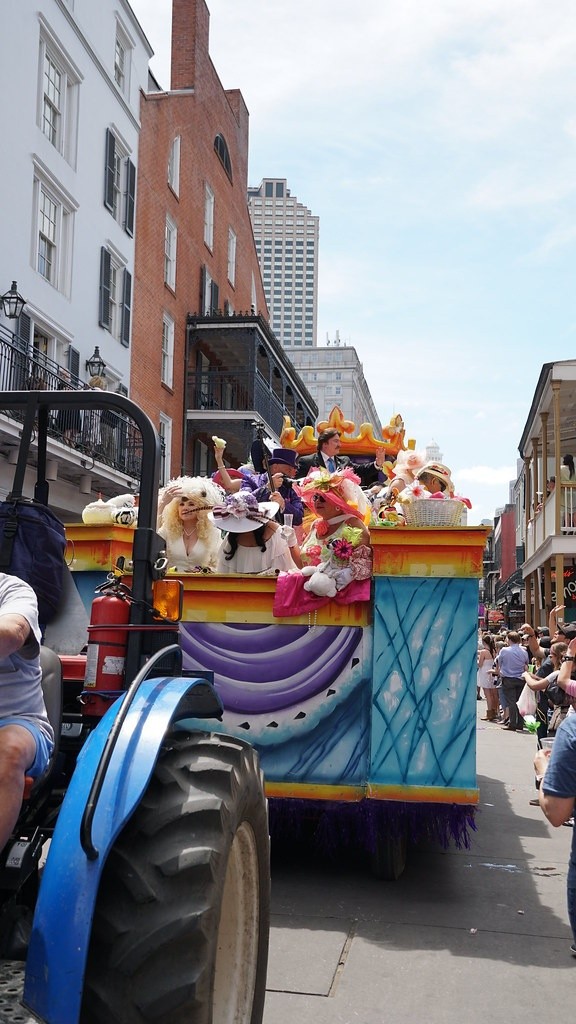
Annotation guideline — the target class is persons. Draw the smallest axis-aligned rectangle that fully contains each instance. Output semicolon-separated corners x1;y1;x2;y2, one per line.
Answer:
54;368;82;444
132;427;454;575
100;389;126;466
532;454;576;535
478;604;576;958
0;571;55;851
76;375;107;457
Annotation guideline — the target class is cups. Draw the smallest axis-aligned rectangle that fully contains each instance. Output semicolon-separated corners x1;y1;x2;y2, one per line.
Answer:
540;737;555;754
284;513;293;528
528;665;535;673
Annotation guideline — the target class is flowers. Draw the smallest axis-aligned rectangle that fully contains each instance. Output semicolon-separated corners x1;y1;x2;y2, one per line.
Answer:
333;538;354;564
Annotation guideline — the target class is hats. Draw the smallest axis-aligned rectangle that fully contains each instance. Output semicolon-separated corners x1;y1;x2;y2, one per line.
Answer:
281;524;297;548
132;485;140;497
268;449;297;467
206;491;280;533
537;626;549;636
395;447;422;470
557;622;576;639
415;460;455;493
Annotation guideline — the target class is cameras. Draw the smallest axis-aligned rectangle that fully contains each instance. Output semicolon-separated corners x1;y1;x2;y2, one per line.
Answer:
281;475;292;488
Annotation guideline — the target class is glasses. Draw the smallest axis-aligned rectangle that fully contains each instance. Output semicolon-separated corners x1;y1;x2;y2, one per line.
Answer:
547;655;552;658
312;495;326;503
439;481;446;492
556;629;562;635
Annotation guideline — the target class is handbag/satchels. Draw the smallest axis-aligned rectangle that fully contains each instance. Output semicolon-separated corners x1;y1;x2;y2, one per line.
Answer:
544;675;565;704
0;388;74;625
516;682;538;716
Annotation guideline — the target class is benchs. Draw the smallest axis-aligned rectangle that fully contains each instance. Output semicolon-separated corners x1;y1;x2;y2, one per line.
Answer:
196;389;220;410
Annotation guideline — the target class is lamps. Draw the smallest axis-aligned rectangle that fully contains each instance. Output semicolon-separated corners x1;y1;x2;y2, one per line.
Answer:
0;281;27;319
8;449;19;464
46;460;59;480
85;345;106;377
80;476;92;495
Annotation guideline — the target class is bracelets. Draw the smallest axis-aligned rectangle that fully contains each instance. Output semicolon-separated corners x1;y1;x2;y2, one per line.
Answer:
562;655;574;661
528;634;535;637
218;465;225;468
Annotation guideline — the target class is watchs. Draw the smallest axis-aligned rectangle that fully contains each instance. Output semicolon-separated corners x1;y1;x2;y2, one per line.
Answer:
534;774;544;790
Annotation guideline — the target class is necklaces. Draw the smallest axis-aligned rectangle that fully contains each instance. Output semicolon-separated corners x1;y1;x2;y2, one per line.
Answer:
182;526;197;540
308;610;317;633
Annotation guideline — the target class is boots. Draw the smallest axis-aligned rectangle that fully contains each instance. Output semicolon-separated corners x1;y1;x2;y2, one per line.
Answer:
481;709;497;721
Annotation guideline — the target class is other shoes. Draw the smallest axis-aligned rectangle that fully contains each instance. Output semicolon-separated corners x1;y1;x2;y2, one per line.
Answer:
497;716;524;731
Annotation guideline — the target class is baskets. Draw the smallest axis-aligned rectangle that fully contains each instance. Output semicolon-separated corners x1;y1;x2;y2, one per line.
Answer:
400;498;465;527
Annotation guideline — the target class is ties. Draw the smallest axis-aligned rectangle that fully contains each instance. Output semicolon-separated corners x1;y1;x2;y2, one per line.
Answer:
327;458;335;473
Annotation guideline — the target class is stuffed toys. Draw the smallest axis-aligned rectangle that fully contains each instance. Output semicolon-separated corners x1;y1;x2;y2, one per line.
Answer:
82;493;138;527
300;562;337;598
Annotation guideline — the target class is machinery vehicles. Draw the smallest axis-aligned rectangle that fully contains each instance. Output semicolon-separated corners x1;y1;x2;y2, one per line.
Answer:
1;390;275;1024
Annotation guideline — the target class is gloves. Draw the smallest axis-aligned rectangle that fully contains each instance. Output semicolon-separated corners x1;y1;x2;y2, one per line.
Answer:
113;509;136;528
333;567;355;591
